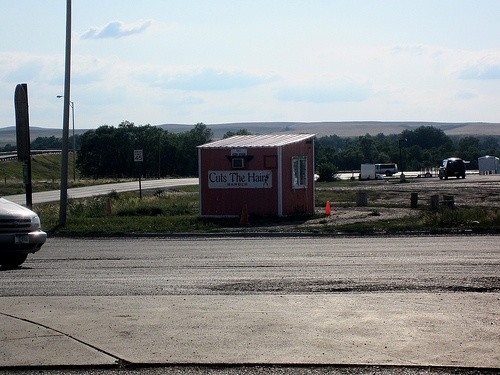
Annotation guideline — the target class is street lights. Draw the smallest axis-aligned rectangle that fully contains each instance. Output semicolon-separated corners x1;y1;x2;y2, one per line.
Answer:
57;95;75;183
399;138;408;171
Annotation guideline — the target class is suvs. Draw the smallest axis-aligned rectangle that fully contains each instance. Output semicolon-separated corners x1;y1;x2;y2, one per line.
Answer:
438;157;467;180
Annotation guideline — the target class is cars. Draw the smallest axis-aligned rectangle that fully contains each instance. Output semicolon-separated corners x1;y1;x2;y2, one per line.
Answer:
0;197;48;269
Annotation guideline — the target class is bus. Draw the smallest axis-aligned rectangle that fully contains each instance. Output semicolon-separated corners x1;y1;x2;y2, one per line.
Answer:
374;163;398;176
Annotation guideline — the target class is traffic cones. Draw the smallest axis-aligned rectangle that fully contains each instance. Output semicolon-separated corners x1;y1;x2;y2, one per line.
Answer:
325;200;331;216
